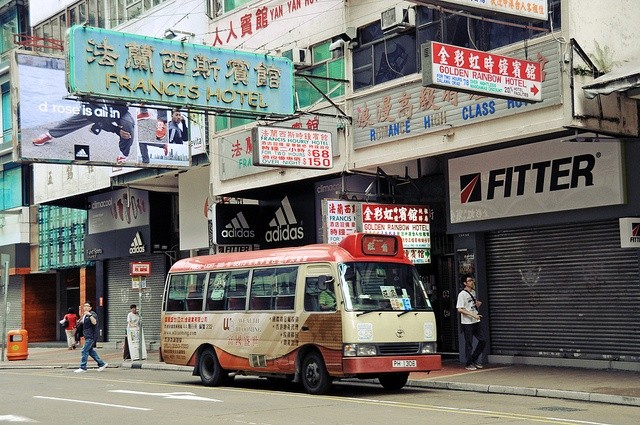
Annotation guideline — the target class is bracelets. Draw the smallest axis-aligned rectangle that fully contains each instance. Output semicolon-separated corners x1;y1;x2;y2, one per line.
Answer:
89;315;93;319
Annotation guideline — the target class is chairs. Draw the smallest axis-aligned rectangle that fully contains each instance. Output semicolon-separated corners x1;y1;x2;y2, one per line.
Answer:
186;291;201;310
209;296;222;311
274;290;292;309
227;293;243;309
251;292;267;309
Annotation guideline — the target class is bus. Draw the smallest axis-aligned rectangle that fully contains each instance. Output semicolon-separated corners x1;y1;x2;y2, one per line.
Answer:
159;233;442;394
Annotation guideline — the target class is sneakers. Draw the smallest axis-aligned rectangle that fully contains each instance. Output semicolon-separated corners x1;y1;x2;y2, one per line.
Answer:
98;363;108;371
464;364;477;371
74;368;87;373
472;361;483;368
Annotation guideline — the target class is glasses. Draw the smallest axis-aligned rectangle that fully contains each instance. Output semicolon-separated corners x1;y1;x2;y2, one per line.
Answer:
465;280;474;282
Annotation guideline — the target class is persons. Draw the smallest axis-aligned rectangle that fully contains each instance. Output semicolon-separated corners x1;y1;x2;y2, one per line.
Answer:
75;300;108;373
455;273;480;370
319;288;338;311
139;144;169;165
32;97;135;164
168;109;188;144
63;307;78;350
127;304;140;327
137;105;168;144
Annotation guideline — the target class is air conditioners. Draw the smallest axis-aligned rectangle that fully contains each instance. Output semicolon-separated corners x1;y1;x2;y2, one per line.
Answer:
282;48;311;66
380;7;416;30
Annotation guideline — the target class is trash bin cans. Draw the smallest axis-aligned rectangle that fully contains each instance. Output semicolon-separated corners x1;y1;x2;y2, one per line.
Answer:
6;329;29;361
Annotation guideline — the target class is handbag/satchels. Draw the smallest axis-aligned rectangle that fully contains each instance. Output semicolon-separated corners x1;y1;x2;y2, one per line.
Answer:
61;314;69;328
464;289;485;320
